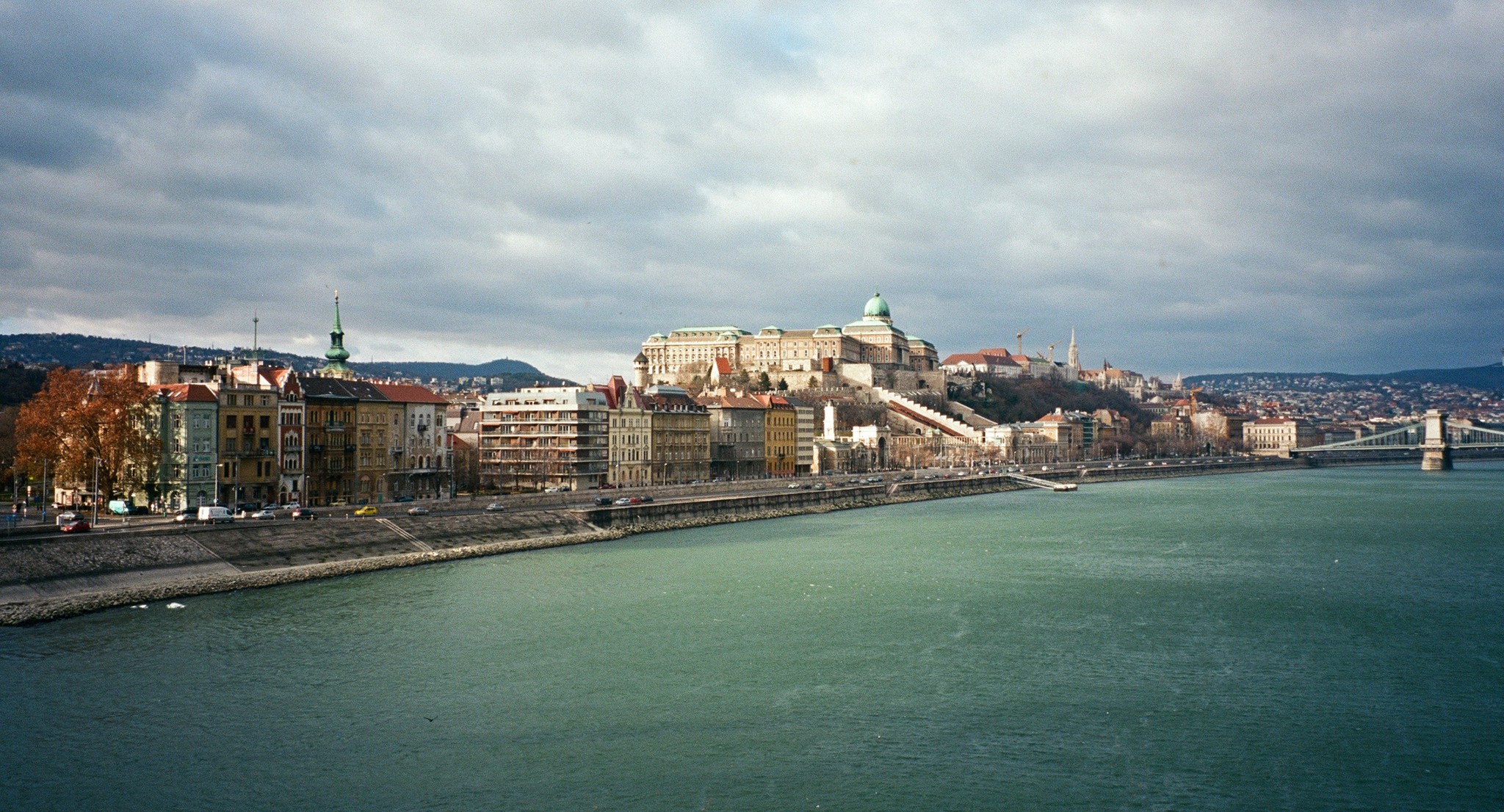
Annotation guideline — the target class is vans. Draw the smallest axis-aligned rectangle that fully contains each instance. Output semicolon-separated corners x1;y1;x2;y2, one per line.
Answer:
108;500;138;515
198;506;235;522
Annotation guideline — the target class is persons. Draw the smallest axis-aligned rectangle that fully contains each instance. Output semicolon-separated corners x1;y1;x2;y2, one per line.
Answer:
151;502;156;515
16;502;65;515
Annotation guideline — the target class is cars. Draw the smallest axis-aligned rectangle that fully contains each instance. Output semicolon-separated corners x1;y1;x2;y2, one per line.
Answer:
174;506;199;522
60;520;91;533
58;511;85;521
354;506;378;516
594;494;653;506
135;506;150;515
487;503;505;511
252;509;276;520
788;442;1279;490
398;496;414;502
544;486;571;492
292;508;320;521
232;501;301;514
598;481;624;490
408;507;430;516
329;499;347;507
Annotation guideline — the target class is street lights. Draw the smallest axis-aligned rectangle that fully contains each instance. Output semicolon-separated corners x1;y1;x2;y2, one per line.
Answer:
231;455;242;515
93;456;110;524
215;464;223;506
664;463;668;486
306;476;310;507
616;465;619;489
692;477;723;484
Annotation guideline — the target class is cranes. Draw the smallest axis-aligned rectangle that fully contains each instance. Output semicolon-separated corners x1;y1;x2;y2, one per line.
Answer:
1016;328;1070;361
